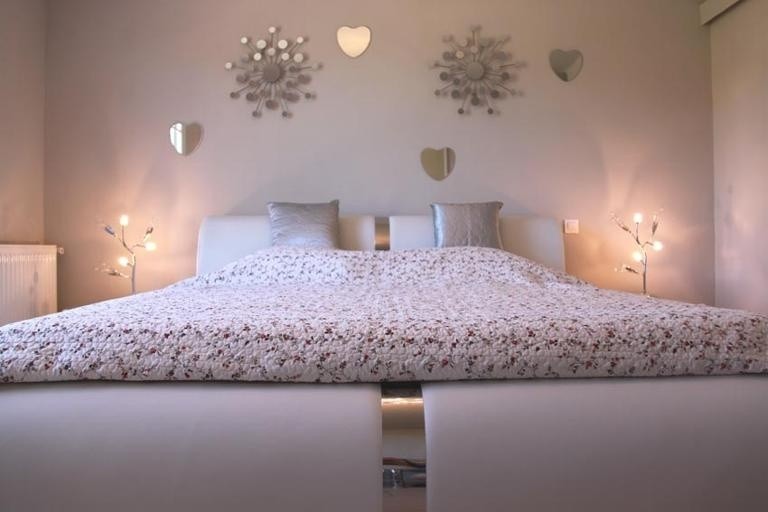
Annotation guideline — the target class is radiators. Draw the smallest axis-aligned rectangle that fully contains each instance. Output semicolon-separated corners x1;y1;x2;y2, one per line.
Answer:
1;244;65;325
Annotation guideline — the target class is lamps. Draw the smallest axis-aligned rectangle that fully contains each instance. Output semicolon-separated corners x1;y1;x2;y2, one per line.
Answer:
608;210;665;293
100;215;156;294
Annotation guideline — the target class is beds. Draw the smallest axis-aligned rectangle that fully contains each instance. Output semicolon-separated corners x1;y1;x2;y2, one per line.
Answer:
1;215;767;512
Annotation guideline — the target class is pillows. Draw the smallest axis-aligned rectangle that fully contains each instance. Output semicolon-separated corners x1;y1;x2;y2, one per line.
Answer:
430;202;502;247
270;202;338;247
